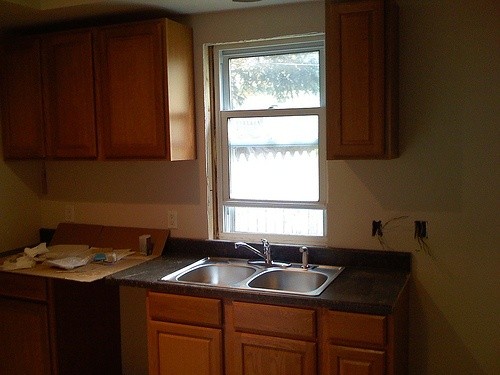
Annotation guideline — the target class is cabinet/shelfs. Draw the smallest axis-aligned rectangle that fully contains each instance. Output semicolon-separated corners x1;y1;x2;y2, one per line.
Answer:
323;308;409;375
0;270;120;375
141;291;225;375
0;33;46;163
99;16;198;164
225;298;323;375
42;27;99;163
324;0;402;162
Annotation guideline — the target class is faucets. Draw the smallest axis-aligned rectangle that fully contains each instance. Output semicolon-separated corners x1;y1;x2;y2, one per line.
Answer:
234;238;274;265
300;246;310;269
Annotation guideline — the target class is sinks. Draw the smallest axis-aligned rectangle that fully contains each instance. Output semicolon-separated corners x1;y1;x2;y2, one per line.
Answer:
244;262;345;296
158;256;265;290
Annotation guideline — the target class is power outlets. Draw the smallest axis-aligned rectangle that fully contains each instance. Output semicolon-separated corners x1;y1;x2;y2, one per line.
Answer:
65;203;75;222
168;210;179;229
